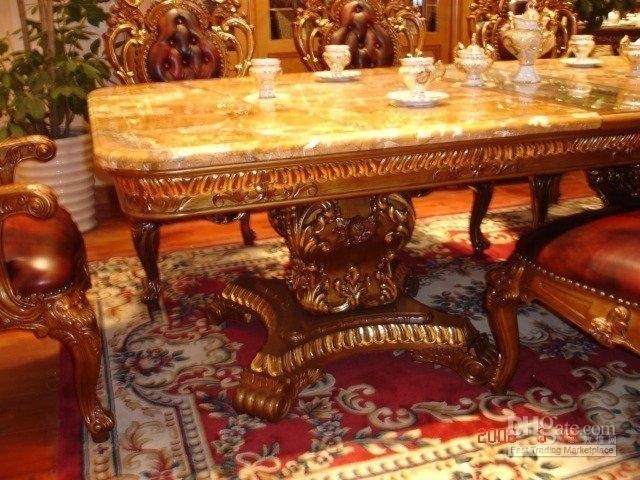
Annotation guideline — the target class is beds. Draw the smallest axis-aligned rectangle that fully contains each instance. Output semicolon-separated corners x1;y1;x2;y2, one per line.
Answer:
86;50;640;420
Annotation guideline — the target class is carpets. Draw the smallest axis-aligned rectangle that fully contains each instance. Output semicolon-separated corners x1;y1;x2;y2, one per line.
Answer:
79;187;640;479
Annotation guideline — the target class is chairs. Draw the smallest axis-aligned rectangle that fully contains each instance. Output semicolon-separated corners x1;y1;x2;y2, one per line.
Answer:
292;0;494;253
102;0;259;307
1;133;116;443
466;0;608;231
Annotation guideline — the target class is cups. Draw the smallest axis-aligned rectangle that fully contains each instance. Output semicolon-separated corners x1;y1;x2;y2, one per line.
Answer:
322;44;350;75
400;53;445;100
249;56;283;98
569;32;594;57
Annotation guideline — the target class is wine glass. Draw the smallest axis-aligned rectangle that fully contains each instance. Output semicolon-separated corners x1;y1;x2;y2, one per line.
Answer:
453;44;494;88
565;71;595;99
501;6;559;85
614;75;640;111
619;37;640;77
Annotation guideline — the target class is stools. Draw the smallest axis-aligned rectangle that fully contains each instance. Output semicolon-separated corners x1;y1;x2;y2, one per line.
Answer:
486;202;640;393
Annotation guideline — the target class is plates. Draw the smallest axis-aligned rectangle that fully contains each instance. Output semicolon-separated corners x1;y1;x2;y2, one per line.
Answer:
385;89;450;106
243;91;292;105
558;57;605;68
313;71;362;81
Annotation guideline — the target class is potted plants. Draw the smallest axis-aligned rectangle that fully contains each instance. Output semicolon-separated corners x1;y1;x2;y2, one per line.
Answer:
0;0;113;238
